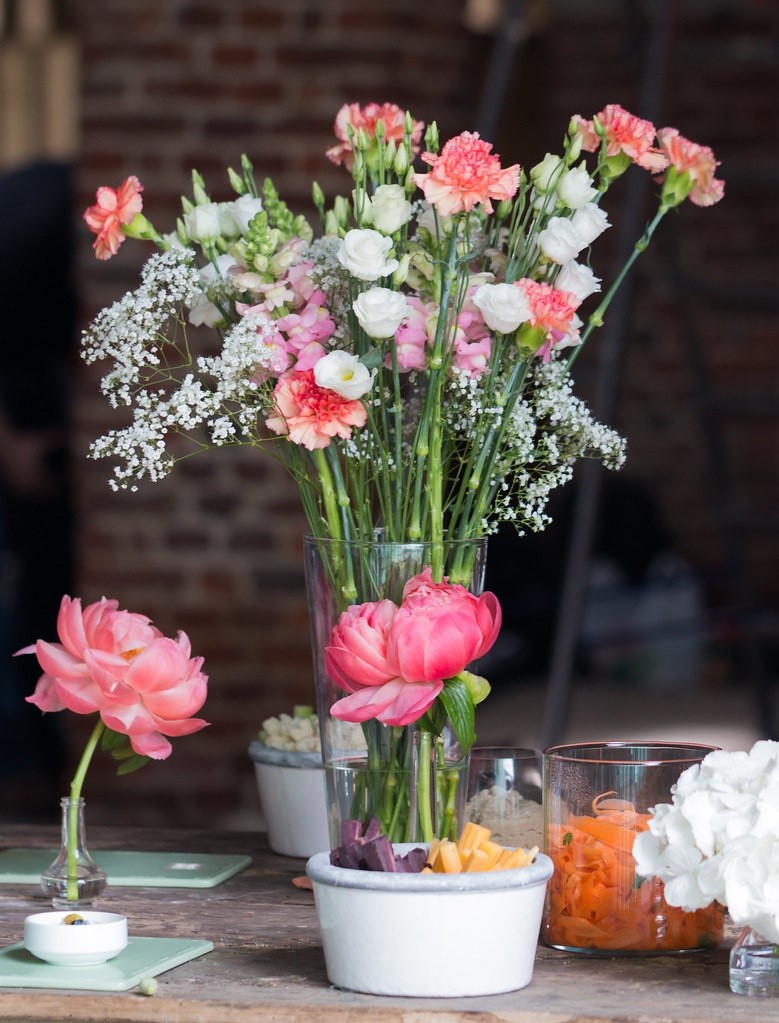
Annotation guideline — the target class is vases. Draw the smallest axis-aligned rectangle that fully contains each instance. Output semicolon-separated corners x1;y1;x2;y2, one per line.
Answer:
248;739;377;858
294;528;489;859
729;926;779;998
39;795;108;910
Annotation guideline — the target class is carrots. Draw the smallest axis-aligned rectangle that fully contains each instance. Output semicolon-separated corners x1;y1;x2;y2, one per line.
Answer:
419;822;541;874
543;791;725;951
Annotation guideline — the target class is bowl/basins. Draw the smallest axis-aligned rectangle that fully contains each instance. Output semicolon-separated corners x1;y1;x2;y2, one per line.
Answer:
469;740;722;958
23;911;129;968
247;737;370;858
305;842;554;998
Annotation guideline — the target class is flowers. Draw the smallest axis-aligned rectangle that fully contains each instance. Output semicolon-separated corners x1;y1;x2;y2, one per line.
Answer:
80;99;728;849
631;737;779;947
13;595;211;896
259;705;367;756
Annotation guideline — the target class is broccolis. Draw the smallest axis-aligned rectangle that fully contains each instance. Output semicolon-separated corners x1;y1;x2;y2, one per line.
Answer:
259;711;370;752
